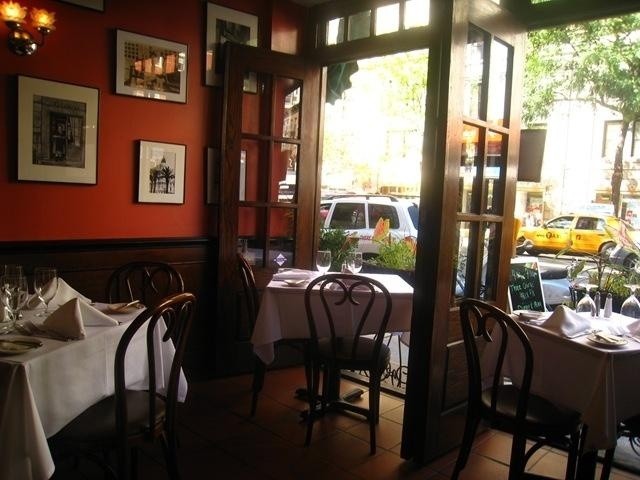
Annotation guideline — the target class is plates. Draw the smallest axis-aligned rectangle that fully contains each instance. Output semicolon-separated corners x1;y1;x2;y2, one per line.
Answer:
1;338;42;355
285;279;307;286
514;309;543;318
589;333;627;347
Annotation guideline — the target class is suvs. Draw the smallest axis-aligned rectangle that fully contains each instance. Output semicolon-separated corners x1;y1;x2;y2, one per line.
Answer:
313;196;419;251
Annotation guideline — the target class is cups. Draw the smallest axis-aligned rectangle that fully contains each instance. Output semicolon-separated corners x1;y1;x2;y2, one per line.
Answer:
2;265;60;335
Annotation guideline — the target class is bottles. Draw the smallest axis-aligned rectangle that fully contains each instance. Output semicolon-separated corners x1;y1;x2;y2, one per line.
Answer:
593;292;602;317
604;293;613;318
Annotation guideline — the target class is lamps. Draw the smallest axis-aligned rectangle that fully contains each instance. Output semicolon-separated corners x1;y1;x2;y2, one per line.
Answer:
0;1;56;57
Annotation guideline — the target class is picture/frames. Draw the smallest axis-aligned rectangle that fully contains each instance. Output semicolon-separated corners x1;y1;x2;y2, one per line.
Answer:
13;72;100;187
134;138;187;206
112;27;189;106
201;2;261;97
204;144;248;207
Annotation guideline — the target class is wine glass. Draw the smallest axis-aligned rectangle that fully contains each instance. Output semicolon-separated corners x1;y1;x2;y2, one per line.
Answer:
347;251;363;274
316;251;332;285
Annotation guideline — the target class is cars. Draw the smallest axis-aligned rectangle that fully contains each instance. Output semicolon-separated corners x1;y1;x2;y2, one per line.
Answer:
277;181;425;213
515;211;620;257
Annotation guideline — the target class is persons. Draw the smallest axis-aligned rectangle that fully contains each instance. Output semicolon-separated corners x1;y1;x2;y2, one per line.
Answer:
53;123;68;160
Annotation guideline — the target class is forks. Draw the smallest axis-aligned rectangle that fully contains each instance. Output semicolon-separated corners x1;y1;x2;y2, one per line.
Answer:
16;321;76;344
